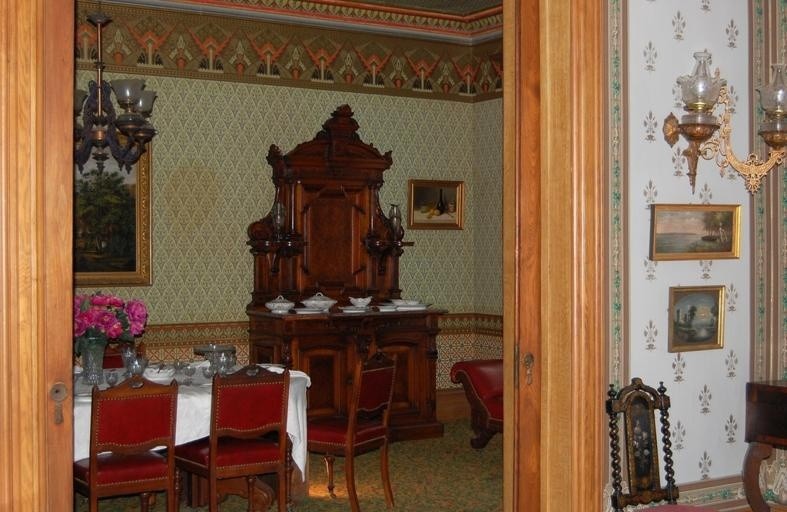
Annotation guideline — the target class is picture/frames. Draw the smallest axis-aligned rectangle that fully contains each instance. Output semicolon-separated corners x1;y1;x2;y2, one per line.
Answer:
406;178;467;230
667;283;726;354
69;126;154;289
647;202;744;262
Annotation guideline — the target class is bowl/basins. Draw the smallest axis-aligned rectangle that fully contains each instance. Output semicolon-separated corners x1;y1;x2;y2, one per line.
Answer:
265;295;429;314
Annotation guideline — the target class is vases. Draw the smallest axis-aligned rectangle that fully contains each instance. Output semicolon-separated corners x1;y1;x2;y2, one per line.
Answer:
80;341;105;386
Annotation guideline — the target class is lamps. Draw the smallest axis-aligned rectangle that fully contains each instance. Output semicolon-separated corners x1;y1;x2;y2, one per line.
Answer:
67;0;162;174
658;46;787;196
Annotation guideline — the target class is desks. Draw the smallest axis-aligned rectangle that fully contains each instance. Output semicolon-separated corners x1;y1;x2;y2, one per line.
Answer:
737;378;787;512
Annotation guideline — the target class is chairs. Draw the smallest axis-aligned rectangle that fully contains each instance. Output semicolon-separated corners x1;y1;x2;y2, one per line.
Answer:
169;358;293;511
73;369;185;511
305;352;399;510
602;374;716;512
448;357;502;450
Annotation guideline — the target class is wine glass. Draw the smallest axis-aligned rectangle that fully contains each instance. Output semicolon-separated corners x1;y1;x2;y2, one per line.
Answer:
107;346;237;387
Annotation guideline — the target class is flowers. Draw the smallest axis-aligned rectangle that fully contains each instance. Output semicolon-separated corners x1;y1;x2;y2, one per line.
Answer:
72;291;147;351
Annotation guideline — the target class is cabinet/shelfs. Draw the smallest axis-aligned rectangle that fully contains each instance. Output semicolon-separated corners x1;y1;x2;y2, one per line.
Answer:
243;98;447;445
